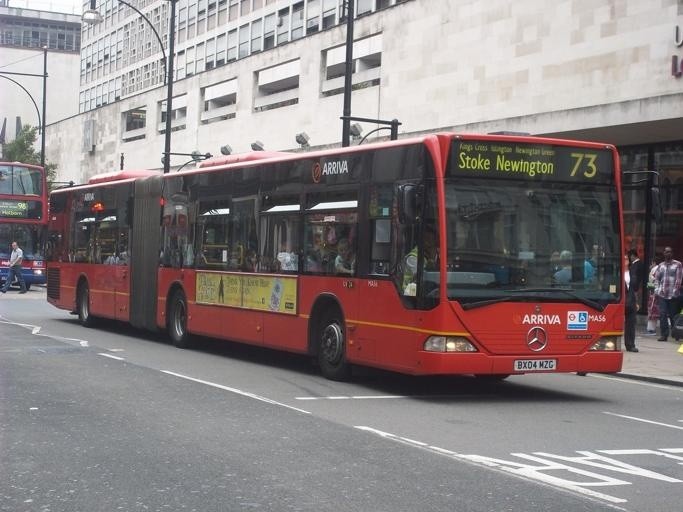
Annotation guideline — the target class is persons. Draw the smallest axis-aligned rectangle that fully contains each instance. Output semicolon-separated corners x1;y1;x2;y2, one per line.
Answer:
623;248;683;355
64;237;595;354
0;241;29;294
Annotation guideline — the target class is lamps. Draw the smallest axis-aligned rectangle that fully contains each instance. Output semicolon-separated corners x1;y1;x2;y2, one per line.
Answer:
249;140;265;151
350;123;366;142
294;131;312;148
219;144;234;155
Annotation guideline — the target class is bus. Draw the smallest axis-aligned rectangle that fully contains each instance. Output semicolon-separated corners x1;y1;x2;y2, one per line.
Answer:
0;160;48;289
39;115;664;382
620;207;683;262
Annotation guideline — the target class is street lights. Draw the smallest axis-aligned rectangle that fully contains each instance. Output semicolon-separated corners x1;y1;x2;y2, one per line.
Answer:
0;45;49;168
81;0;179;175
